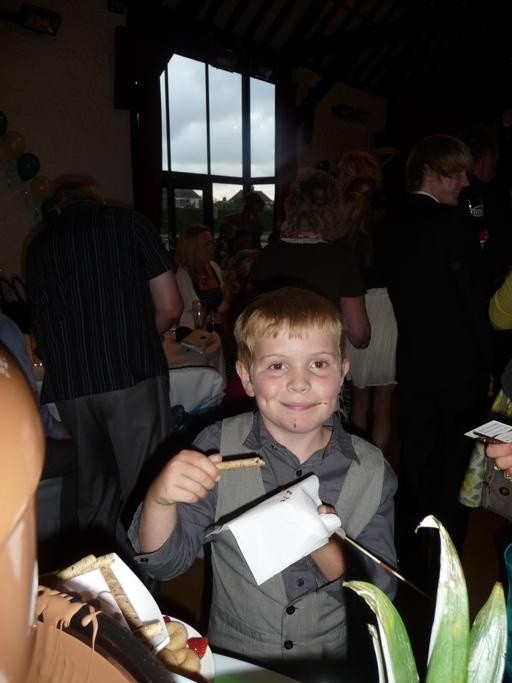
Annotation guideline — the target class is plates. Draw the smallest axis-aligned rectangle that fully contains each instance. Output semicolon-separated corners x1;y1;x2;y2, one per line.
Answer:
161;614;215;683
38;553;171;657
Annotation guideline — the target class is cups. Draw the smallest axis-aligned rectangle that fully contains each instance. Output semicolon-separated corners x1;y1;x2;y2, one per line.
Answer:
463;197;487;243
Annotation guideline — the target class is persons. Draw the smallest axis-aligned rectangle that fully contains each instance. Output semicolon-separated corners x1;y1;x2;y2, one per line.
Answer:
0;132;512;682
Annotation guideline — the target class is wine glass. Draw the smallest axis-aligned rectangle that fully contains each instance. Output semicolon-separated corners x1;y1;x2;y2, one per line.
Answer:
192;301;208;329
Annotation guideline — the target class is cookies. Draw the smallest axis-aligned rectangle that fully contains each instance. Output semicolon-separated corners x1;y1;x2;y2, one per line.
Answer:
56;552;162;649
213;458;266;471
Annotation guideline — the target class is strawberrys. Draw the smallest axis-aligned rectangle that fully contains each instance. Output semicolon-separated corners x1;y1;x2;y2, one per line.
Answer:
187;637;208;659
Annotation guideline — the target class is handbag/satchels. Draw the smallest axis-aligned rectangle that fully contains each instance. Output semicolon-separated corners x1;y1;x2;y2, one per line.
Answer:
482;467;512;523
